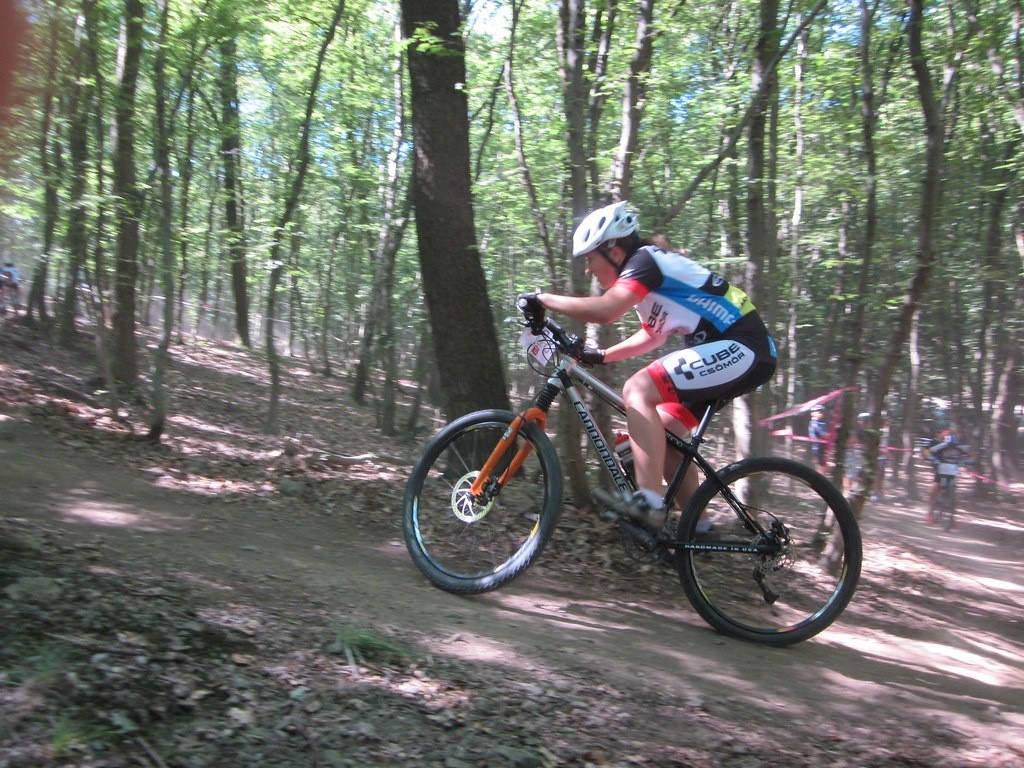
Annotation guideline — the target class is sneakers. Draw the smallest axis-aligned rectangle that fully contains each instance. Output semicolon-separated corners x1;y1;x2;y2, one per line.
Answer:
590;487;668;535
692;524;722;545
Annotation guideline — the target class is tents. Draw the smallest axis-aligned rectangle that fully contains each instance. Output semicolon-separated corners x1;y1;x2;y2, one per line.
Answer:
758;385;860;440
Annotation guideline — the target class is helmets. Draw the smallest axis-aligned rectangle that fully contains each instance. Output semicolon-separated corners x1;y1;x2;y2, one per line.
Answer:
810;404;825;411
571;200;639;258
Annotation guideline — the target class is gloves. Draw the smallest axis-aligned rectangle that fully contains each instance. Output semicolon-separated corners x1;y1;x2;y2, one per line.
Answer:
516;292;546;317
577;346;606;367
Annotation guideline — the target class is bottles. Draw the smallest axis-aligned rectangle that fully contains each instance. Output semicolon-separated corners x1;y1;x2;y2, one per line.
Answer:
614;431;639;492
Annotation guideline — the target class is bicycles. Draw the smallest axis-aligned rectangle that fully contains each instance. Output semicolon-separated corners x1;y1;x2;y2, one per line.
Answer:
926;476;957;532
402;295;863;647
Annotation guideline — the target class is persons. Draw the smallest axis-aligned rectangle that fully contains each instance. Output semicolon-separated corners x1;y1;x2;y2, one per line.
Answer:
2;260;21;307
516;199;777;554
920;424;976;529
808;407;890;543
